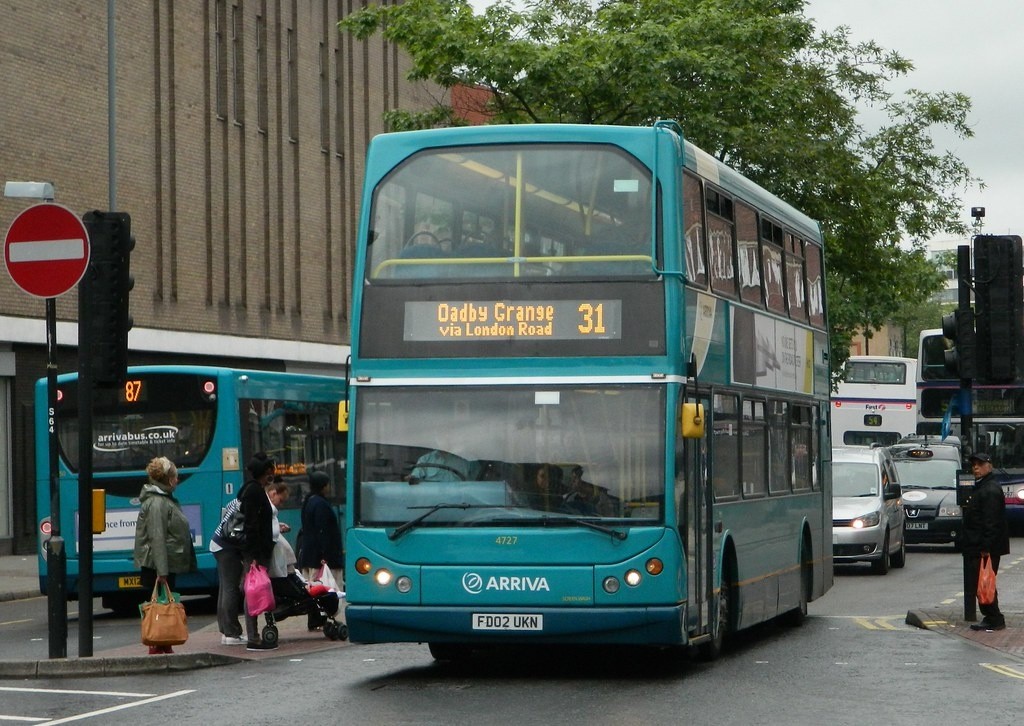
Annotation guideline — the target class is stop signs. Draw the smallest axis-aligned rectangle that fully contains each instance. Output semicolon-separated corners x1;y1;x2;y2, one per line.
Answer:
2;201;90;299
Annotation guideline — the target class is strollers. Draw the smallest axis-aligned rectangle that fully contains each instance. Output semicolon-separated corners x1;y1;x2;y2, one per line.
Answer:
242;560;348;641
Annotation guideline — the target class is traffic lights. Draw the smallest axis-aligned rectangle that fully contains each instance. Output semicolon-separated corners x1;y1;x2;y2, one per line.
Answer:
79;209;134;386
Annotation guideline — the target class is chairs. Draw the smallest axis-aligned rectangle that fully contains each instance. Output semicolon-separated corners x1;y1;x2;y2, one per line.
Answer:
399;228;498;262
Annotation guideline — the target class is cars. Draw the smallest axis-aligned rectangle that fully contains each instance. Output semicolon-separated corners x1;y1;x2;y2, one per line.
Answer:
898;433;962;452
889;444;965;552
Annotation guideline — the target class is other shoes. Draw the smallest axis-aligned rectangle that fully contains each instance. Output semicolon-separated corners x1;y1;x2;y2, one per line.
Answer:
971;612;1006;630
246;639;280;651
221;633;248;644
338;592;346;599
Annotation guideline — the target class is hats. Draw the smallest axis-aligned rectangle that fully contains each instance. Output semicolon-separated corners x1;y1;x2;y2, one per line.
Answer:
969;452;993;464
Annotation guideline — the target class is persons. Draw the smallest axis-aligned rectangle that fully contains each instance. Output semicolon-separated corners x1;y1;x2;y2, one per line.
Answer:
208;452;347;652
400;419;482;484
956;452;1011;631
134;455;199;655
530;418;628;520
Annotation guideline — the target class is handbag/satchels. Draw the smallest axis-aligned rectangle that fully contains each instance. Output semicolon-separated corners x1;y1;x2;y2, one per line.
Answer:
244;562;276;617
220;482;262;550
977;555;996;604
267;538;288;577
314;562;343;615
138;574;189;645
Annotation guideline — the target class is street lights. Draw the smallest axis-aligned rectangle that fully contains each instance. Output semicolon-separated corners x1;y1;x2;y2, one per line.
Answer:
3;181;68;658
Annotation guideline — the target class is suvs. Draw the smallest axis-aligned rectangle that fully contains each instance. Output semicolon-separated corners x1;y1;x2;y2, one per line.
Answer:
832;441;906;575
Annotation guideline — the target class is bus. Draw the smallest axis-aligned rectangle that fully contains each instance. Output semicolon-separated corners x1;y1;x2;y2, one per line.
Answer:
830;357;916;446
34;365;348;619
335;120;832;663
916;328;1024;537
